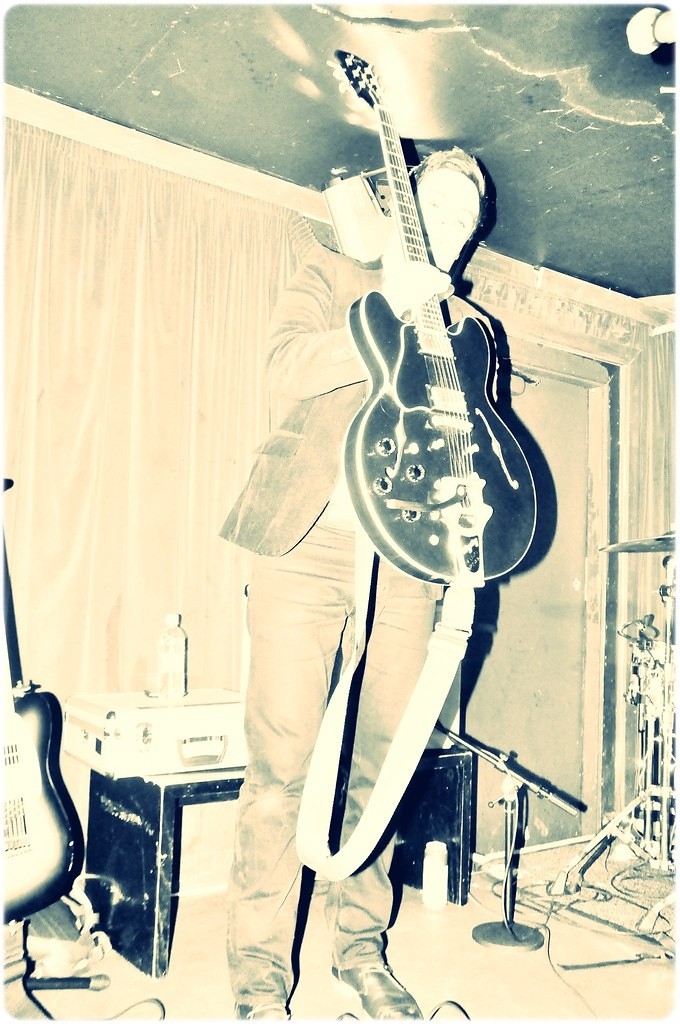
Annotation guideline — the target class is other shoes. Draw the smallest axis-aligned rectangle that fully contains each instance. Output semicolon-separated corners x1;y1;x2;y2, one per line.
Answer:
332;962;424;1024
244;1001;288;1024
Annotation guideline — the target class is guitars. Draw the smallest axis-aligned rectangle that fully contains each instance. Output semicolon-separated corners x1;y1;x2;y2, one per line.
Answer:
1;477;90;927
326;40;551;592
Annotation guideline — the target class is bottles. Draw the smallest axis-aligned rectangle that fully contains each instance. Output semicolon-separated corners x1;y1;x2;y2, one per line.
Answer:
146;613;188;700
423;841;451;908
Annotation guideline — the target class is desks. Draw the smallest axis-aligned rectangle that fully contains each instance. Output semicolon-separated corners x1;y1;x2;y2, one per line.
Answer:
84;740;474;976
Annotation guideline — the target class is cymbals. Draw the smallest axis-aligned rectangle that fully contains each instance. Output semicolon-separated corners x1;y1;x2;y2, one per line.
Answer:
598;528;677;554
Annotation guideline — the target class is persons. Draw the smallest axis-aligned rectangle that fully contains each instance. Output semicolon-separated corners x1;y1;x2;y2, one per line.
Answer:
219;148;498;1024
